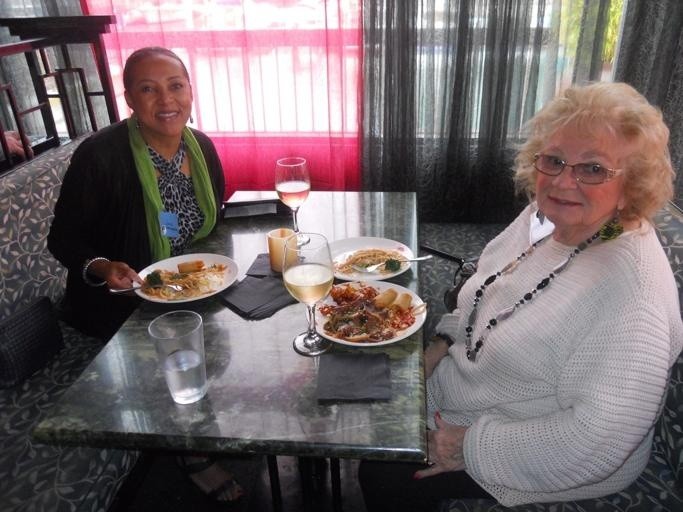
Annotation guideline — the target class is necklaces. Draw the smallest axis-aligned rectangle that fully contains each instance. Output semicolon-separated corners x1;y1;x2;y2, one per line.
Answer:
463;212;623;363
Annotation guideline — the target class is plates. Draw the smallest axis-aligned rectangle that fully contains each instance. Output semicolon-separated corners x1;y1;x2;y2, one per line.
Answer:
304;280;429;349
134;254;240;303
330;235;412;280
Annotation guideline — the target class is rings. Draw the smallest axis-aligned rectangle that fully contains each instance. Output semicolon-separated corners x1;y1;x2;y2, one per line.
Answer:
427;460;434;466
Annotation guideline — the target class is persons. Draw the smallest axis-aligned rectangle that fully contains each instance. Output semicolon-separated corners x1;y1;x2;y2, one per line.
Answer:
356;78;683;511
49;46;249;505
1;127;35;161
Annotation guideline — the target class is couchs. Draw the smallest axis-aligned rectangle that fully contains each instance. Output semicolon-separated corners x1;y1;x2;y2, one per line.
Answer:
1;132;140;512
414;200;682;512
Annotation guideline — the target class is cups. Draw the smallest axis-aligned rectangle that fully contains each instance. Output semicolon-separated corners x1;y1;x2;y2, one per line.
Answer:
148;309;209;405
268;229;299;271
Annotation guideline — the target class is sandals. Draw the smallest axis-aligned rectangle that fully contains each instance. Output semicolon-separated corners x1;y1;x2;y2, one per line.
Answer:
179;452;245;503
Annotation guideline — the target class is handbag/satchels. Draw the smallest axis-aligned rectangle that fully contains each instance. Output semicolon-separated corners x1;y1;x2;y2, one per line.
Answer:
444;262;478;314
0;295;65;390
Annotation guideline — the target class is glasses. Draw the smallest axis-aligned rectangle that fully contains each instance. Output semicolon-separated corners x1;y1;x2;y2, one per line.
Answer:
531;152;624;186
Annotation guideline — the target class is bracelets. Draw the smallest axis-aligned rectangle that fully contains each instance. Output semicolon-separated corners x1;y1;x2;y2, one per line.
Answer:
434;332;453;349
82;256;112;287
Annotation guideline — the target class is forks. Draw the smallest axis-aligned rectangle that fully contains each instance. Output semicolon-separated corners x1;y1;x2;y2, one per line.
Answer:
346;253;431;273
109;281;189;294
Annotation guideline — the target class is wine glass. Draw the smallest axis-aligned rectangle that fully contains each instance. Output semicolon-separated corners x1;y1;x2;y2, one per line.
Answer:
279;232;335;357
275;159;313;244
